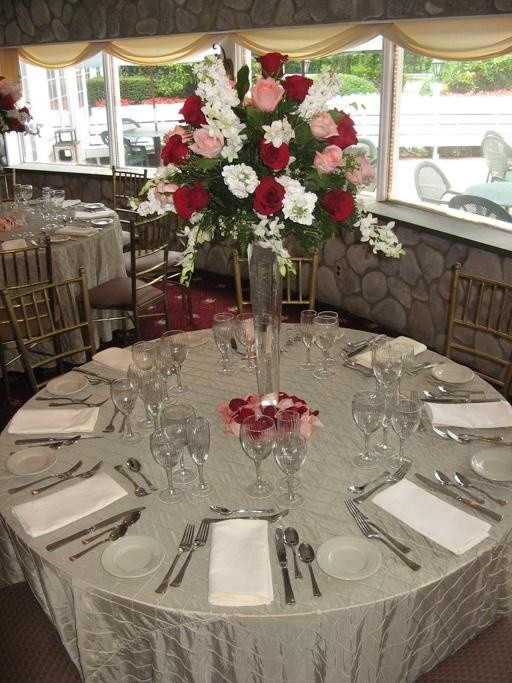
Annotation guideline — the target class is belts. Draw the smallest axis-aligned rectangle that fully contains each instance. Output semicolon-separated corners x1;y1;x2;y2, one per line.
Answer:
2;183;77;231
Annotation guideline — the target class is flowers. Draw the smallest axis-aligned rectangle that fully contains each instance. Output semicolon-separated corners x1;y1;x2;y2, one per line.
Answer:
134;50;408;287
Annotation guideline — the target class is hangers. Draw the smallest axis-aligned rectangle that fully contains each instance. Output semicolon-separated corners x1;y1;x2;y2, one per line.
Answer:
44;508;143;561
285;525;326;606
424;383;485;399
415;466;508;524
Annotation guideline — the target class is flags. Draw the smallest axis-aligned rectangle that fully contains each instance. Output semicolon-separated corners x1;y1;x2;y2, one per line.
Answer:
245;237;287;412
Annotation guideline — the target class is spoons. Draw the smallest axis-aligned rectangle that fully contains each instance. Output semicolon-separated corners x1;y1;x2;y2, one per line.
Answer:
348;471;390;494
44;508;143;561
415;466;508;524
126;456;157;493
424;383;485;399
285;525;326;606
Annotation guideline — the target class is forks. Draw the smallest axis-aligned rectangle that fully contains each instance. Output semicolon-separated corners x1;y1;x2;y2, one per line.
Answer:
9;367;117;495
156;519;211;594
431;426;511;445
342;334;423;383
354;462;411;503
103;401;127;436
345;500;419;575
202;509;290;525
114;464;146;498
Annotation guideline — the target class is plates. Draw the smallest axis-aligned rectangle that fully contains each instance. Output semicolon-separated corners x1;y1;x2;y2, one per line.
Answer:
470;448;512;481
83;201;116;229
102;532;167;577
315;536;384;583
431;361;475;384
47;233;71;243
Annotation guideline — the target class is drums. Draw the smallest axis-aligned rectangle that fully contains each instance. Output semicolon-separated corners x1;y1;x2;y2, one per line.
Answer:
29;240;44;253
274;526;295;606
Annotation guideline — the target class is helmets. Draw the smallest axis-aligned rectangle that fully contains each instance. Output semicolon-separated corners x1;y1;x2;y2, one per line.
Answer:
83;201;116;229
470;448;512;481
47;233;71;243
431;361;475;384
315;536;384;583
102;532;167;577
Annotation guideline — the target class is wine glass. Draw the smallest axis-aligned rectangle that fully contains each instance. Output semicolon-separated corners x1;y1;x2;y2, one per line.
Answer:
301;310;339;380
2;183;77;231
391;399;421;469
274;435;304;506
109;328;210;495
352;390;380;469
239;414;274;499
277;412;303;491
374;392;399;457
212;313;260;376
371;339;415;402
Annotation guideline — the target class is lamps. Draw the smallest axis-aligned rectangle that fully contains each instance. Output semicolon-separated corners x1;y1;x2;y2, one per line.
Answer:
100;117;168;166
229;246;320;325
413;129;511;222
445;260;512;398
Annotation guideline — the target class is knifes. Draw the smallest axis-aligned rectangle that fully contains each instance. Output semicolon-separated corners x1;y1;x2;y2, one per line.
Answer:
274;526;295;606
29;240;44;253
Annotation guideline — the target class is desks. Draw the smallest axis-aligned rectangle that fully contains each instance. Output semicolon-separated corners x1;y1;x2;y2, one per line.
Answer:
431;57;445;163
300;59;312;76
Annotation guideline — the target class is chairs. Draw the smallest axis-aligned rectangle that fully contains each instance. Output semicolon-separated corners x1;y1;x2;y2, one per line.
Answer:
0;163;194;392
413;129;511;222
229;246;320;325
100;117;168;166
445;260;512;398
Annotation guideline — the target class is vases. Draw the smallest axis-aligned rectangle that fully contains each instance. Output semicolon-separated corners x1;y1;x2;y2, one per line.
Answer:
245;237;287;412
134;50;408;287
